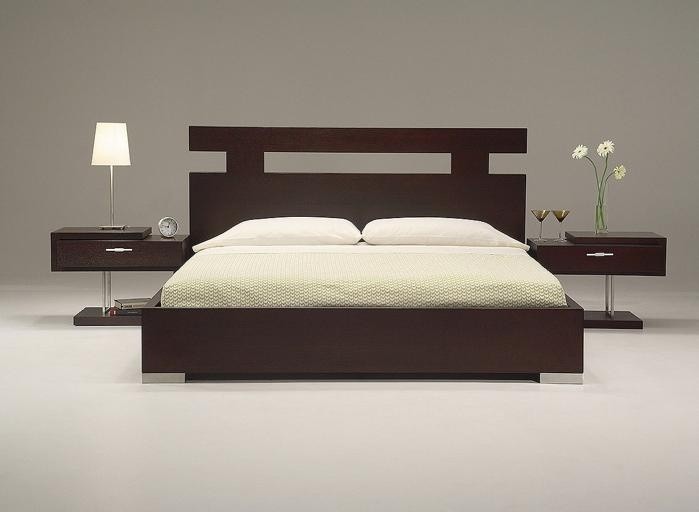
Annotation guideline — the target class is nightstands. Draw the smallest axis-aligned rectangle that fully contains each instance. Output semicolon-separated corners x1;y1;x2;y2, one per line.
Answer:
527;231;668;329
50;226;187;326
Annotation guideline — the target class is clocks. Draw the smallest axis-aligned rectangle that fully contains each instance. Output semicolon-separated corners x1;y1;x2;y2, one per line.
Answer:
157;216;179;238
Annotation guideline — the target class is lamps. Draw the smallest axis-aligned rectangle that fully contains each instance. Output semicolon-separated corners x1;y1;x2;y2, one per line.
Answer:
91;123;131;230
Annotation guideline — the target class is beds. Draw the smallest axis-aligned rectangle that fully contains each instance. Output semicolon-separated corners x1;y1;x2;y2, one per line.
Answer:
141;126;584;383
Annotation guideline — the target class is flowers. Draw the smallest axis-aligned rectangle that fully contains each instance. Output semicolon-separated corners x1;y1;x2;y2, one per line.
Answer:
571;140;626;230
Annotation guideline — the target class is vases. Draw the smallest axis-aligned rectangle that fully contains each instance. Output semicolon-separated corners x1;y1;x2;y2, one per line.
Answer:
594;203;609;232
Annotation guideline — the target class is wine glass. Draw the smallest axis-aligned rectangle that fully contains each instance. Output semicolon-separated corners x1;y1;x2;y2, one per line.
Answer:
529;209;571;242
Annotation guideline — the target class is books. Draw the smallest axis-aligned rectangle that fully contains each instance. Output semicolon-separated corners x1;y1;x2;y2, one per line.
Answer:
108;298;153;316
